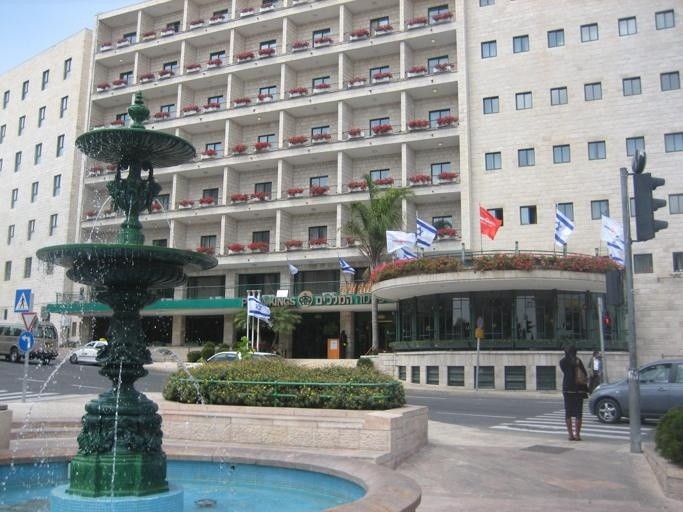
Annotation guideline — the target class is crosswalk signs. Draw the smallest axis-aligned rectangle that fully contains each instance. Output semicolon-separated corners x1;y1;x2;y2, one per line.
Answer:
14;289;31;312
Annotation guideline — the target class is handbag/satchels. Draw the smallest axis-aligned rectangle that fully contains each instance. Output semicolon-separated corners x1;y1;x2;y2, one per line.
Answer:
574;366;588;387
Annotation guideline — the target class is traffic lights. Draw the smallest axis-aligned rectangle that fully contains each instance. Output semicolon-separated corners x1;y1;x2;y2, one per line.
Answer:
633;172;668;242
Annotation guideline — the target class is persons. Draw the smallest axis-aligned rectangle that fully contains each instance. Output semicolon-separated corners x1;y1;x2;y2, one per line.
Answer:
338;330;347;359
558;343;587;441
587;350;602;397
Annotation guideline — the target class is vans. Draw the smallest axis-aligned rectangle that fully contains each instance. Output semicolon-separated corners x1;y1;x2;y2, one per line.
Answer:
0;321;59;365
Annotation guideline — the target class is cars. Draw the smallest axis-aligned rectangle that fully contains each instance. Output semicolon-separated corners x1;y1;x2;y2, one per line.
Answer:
69;340;109;366
588;359;683;424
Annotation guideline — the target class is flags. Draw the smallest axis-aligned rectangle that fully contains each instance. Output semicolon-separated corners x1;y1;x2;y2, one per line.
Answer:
338;257;355;275
247;295;271;319
287;264;298;275
385;207;624;270
